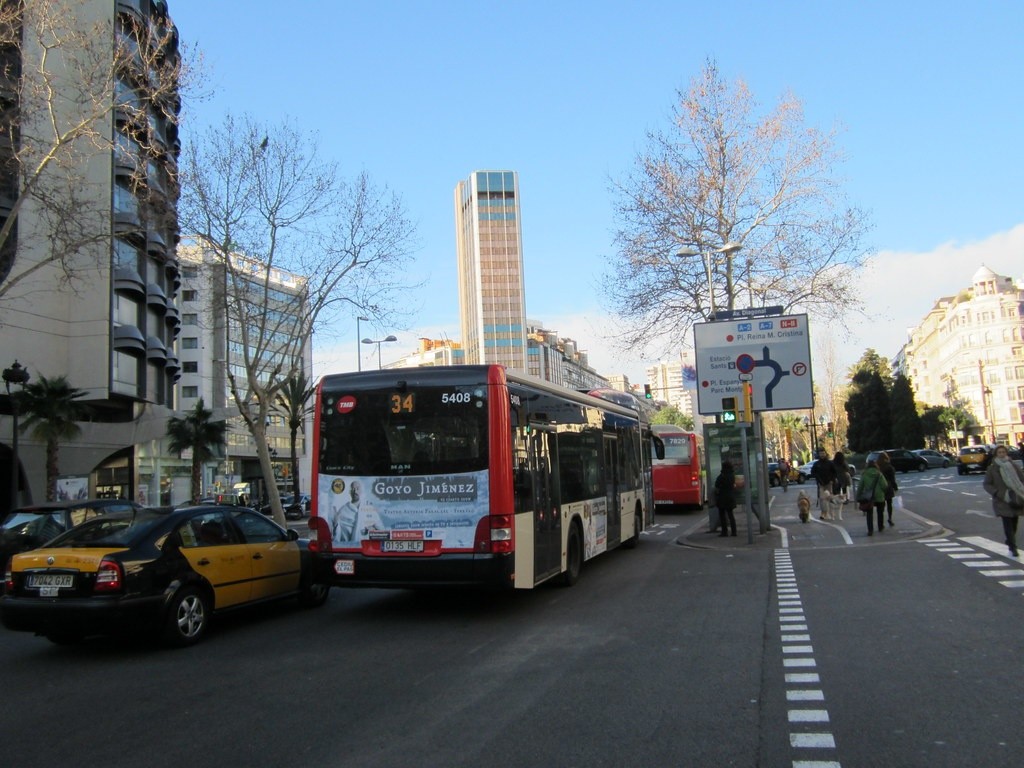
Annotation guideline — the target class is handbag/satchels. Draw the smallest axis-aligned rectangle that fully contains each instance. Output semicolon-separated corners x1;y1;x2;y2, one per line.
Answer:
892;491;902;510
859;500;874;513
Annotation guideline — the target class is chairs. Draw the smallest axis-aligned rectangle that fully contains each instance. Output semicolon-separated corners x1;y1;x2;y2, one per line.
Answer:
198;521;226;542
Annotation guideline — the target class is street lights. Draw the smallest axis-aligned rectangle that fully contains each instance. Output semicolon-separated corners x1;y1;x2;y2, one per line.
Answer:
676;241;743;310
2;359;31;508
361;336;397;370
357;316;368;371
984;387;995;445
803;415;824;459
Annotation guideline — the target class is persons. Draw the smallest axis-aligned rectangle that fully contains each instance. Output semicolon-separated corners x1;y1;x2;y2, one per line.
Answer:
856;460;888;536
715;461;738;537
877;452;898;526
779;458;788;492
333;481;386;543
811;447;850;520
983;445;1024;556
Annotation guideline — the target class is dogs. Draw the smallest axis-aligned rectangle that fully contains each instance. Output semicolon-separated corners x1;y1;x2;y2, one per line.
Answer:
819;486;847;521
797;489;811;524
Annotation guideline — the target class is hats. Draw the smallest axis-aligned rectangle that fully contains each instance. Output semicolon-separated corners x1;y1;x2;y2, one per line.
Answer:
995;445;1007;455
879;451;889;461
866;454;878;464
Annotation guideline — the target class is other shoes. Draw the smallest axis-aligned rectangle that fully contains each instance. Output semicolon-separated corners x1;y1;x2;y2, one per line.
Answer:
718;532;729;537
887;519;894;527
878;526;884;532
867;531;874;537
731;533;737;537
1009;545;1019;557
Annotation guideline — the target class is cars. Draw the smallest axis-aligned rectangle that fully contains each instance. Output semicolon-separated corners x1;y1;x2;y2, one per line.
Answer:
957;444;1020;475
797;460;856;480
866;449;928;473
767;463;806;487
910;450;950;469
0;499;141;591
0;494;334;645
259;495;311;520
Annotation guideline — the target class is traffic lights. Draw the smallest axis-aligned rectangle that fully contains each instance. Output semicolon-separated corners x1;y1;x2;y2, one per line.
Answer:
743;383;753;422
644;384;651;399
722;396;739;423
827;421;834;436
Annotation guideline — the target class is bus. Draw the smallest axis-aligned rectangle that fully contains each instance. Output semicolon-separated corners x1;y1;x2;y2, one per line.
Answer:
309;364;666;589
648;425;707;511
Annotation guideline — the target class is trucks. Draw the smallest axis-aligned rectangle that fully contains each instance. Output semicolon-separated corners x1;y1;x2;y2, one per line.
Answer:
234;483;250;496
